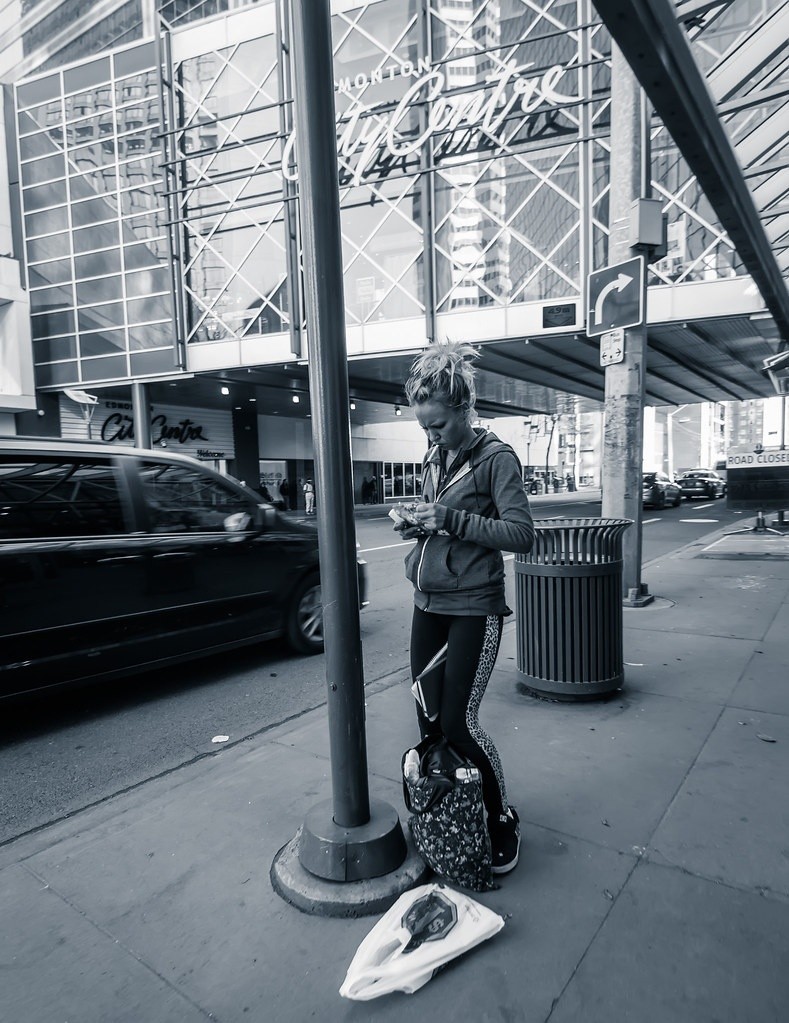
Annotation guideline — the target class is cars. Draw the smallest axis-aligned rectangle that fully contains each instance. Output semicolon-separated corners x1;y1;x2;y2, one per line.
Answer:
642;471;683;512
673;470;728;501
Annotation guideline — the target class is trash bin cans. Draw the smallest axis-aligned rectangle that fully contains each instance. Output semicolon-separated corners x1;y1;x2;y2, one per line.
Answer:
513;516;636;702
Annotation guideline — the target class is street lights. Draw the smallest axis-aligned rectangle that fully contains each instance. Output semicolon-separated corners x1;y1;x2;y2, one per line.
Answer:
63;388;100;440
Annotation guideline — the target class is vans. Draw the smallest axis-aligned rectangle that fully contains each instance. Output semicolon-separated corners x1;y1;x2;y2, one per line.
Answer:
0;434;377;729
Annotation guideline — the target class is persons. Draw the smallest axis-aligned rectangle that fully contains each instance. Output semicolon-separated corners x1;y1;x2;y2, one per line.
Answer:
361;476;370;504
410;475;419;494
371;475;381;505
302;478;316;515
540;469;576;492
280;478;291;511
260;482;272;502
392;475;401;495
389;336;536;874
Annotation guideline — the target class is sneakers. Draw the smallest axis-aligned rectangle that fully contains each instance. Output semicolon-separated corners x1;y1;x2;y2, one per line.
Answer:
486;805;521;874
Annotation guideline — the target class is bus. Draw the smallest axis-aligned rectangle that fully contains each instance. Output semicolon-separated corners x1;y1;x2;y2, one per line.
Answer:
713;456;728;485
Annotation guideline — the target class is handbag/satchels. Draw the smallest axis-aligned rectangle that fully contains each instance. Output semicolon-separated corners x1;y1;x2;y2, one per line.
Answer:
337;882;506;1002
400;733;504;894
